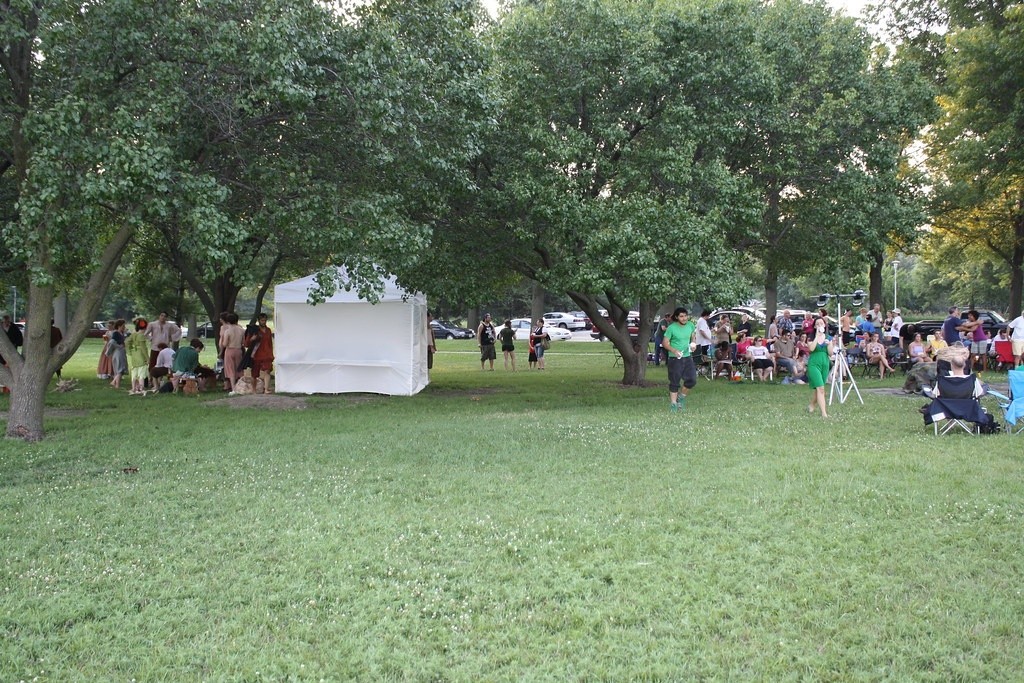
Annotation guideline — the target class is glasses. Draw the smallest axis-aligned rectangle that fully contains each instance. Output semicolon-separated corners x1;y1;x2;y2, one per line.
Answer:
757;340;763;342
786;335;791;337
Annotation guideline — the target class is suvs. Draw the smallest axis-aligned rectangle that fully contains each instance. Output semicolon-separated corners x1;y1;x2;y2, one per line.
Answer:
88;321;132;339
772;312;855;345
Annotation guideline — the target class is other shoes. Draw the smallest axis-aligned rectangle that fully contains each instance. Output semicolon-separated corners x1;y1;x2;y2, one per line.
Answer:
153;389;159;396
489;368;494;371
481;368;486;371
537;368;541;371
228;390;236;396
263;389;271;394
889;368;895;372
541;368;545;372
109;382;116;387
655;363;660;366
115;386;123;390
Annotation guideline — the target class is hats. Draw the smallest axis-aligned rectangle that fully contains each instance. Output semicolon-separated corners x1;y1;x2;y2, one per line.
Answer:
892;308;901;313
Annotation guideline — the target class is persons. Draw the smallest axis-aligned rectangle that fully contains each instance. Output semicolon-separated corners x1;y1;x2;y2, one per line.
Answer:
932;357;983;399
427;312;436;369
662;307;697;408
99;311;274;393
499;319;517;371
477;313;496;371
0;312;23;367
51;318;63;379
528;319;549;370
807;316;833;417
654;304;1023;381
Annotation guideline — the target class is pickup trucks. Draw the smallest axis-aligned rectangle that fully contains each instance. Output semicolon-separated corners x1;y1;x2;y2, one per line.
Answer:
911;308;1019;344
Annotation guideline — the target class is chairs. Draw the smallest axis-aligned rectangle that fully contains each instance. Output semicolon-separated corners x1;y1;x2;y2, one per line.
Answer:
923;374;987;435
694;341;799;381
987;370;1024;434
861;352;891;379
612;344;625;367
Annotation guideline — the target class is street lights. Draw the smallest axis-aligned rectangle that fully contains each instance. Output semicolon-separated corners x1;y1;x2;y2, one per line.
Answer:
11;284;18;324
891;259;900;309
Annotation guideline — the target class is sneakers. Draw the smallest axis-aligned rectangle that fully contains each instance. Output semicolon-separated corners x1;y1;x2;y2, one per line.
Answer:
669;403;678;415
677;395;686;412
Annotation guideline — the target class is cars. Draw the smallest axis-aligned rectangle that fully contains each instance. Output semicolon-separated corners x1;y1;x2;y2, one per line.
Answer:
493;318;572;342
430;319;476;340
540;312;586;332
568;310;593;330
590;317;649;342
702;311;760;332
195;321;214;339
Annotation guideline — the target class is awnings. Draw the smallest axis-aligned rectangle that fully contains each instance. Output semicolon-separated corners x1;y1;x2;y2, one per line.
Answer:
273;251;428;396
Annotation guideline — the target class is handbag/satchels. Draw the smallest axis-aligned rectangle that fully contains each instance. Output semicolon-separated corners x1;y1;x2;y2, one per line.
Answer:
541;336;551;350
